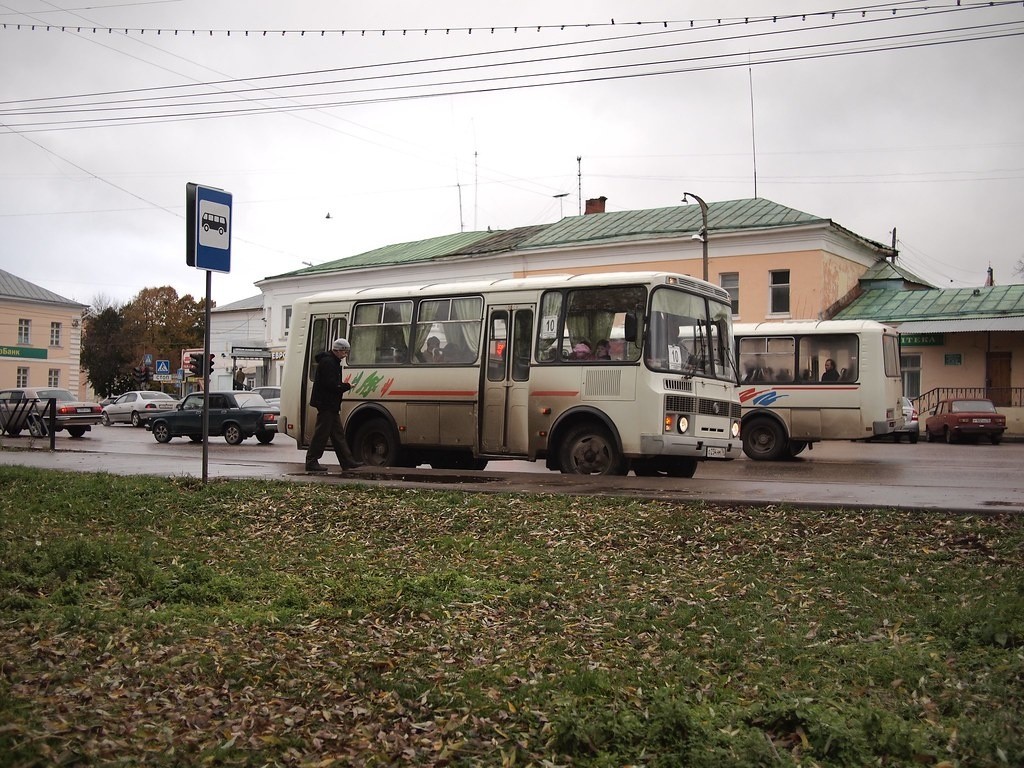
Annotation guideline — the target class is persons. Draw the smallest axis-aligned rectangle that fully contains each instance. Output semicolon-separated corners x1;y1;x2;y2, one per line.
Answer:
237;368;245;390
821;359;840;381
306;337;364;471
744;360;792;383
572;339;610;360
423;336;461;361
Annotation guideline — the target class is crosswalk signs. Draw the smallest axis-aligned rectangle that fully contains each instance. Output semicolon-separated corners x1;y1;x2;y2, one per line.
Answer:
156;360;169;374
177;370;184;380
145;355;152;365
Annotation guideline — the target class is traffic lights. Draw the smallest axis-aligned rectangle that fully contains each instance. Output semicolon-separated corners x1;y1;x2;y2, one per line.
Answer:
190;353;215;377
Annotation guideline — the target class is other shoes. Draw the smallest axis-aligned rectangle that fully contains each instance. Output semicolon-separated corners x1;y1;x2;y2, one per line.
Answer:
306;460;328;471
341;461;364;471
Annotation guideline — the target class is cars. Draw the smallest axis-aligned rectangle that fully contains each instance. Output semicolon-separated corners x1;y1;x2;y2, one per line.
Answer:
925;398;1007;445
180;394;203;406
101;391;179;427
144;390;276;445
101;395;120;405
251;387;281;412
0;387;102;438
899;397;920;444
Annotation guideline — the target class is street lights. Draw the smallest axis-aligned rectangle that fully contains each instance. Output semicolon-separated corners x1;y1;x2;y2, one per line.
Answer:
681;192;708;283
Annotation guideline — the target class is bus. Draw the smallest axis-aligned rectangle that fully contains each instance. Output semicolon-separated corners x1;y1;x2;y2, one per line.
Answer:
266;271;745;478
545;319;905;459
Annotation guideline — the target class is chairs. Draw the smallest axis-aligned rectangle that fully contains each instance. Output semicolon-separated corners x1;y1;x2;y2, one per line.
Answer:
748;368;849;382
448;346;476;364
627;338;643;362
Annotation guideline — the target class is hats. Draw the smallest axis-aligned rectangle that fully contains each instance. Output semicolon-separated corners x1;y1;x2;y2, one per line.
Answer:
573;344;590;354
332;339;350;351
